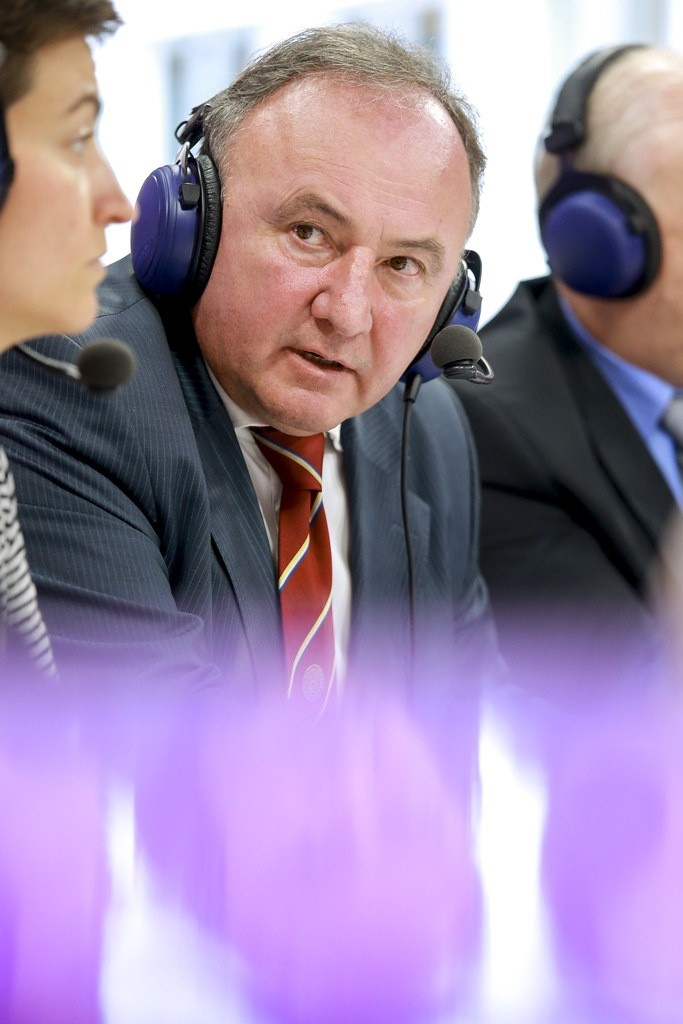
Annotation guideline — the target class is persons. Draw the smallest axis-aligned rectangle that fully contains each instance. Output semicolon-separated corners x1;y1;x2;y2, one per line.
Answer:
0;1;489;734
445;41;683;687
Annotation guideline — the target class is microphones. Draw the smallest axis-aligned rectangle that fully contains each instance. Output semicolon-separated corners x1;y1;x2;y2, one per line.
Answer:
429;324;495;384
15;336;138;393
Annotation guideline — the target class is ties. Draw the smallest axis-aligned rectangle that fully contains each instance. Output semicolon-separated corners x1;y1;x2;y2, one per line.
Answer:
247;426;336;734
657;398;683;480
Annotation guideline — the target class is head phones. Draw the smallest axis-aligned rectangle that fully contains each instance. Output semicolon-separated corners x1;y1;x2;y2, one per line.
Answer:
535;42;663;304
130;95;483;384
